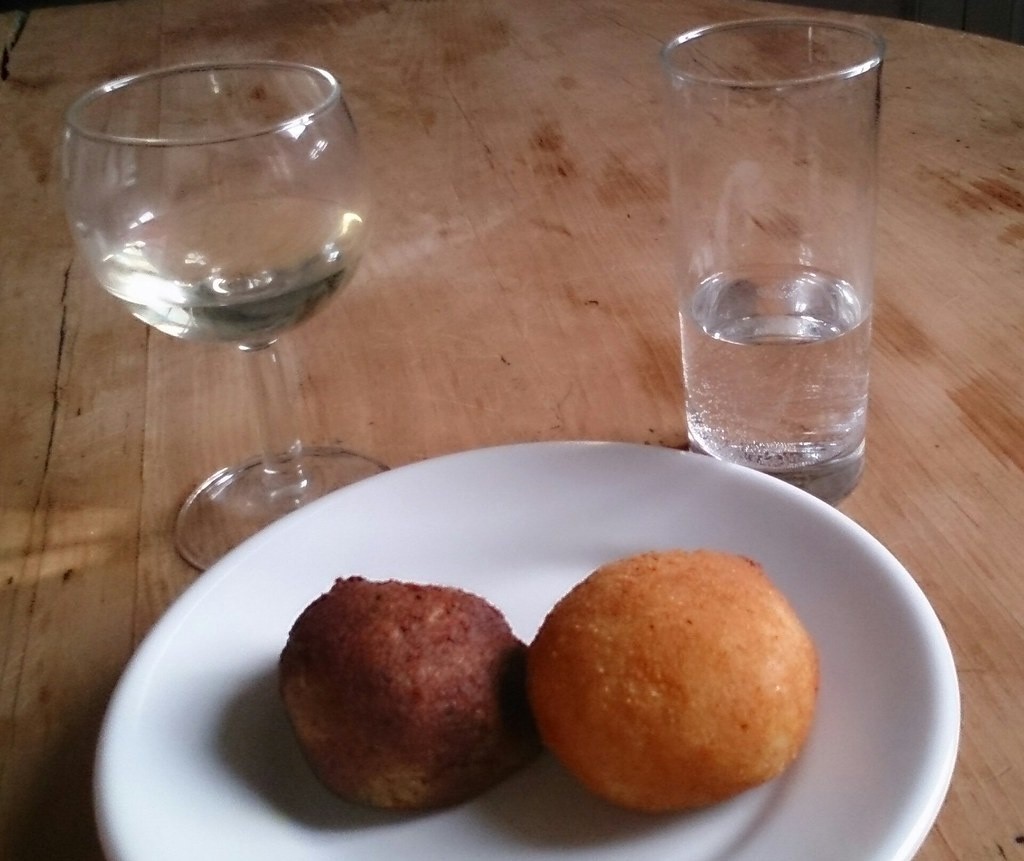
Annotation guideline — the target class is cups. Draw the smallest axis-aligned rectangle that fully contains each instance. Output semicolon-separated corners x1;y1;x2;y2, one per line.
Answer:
661;15;886;509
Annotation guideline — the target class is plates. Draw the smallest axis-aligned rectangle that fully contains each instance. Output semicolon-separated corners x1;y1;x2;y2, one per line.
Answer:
95;438;960;861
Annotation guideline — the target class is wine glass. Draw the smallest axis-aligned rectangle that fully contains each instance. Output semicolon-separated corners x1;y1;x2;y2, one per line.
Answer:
60;61;385;572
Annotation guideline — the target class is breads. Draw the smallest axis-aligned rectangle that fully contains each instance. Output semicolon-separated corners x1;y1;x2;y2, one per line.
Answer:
278;574;538;816
523;549;818;815
